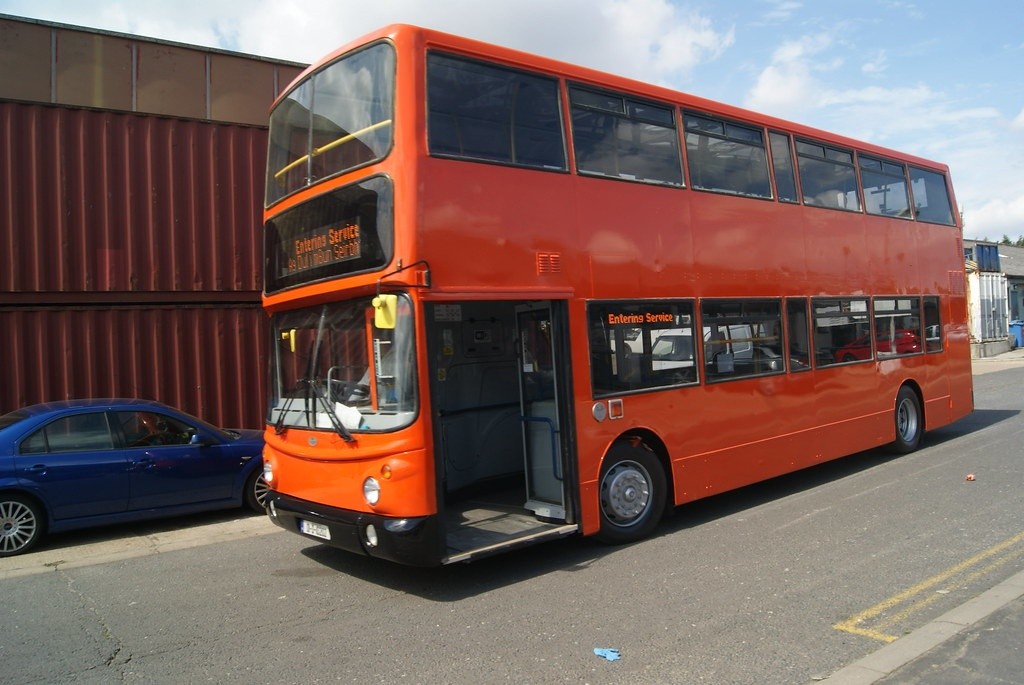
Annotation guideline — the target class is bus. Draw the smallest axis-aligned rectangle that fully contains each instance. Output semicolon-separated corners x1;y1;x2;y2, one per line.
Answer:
256;23;978;574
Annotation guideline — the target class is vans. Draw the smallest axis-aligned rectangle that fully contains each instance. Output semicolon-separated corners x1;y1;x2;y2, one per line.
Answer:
638;321;755;373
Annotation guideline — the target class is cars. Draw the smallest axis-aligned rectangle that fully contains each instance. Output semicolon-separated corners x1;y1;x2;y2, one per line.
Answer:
0;395;274;557
733;324;943;374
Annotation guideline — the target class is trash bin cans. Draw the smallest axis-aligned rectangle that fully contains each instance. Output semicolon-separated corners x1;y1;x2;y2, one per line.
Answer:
1008;319;1024;347
